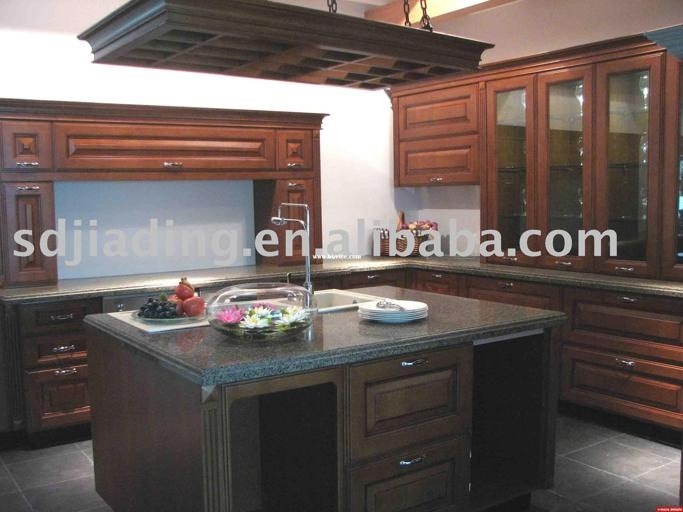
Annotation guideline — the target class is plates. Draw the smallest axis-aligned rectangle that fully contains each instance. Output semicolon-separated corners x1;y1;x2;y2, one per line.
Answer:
357;300;428;324
133;310;207;324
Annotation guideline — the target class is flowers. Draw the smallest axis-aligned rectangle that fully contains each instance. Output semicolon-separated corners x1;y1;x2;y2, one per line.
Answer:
212;302;316;337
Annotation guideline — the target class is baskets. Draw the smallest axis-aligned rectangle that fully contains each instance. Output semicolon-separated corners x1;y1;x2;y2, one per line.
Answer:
373;227;430;257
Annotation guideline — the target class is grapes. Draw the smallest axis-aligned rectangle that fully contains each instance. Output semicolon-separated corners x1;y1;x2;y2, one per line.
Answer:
137;297;176;318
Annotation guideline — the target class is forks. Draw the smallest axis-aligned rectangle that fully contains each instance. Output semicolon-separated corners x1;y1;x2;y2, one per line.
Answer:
376;299;404;311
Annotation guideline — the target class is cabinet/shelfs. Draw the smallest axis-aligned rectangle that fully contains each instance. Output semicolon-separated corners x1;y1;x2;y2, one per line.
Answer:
1;295;104;440
342;343;475;511
480;32;683;282
285;268;399;292
384;63;486;188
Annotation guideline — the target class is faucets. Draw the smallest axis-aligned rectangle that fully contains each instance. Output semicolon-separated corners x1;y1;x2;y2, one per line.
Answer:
269;201;316;310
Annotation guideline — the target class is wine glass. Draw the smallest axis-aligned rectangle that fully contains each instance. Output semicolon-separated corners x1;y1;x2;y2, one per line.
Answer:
518;74;648;220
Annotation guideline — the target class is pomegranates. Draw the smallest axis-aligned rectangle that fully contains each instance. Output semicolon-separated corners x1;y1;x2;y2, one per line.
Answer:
166;277;205;316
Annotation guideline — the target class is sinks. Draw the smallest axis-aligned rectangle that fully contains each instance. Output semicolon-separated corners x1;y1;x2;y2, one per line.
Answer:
274;287;392;313
208;299;312;323
